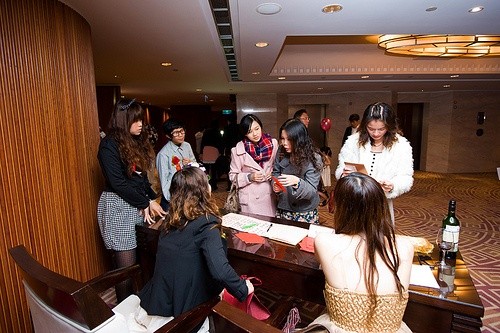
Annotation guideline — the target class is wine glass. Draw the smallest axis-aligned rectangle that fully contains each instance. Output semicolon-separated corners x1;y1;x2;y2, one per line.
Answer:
435;228;454;269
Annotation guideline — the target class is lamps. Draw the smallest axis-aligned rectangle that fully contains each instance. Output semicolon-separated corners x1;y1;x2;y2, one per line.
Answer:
378;34;500;58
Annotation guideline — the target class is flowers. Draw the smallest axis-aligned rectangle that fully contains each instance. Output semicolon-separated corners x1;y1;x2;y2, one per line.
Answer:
172;156;181;171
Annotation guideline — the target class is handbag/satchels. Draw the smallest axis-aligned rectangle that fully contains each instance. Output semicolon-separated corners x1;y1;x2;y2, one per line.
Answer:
224;174;239;214
328;189;336;213
282;307;301;333
319;180;330;207
223;275;272;320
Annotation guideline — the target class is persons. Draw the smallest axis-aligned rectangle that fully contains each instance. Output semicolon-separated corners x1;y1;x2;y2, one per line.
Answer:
341;114;361;147
194;115;245;192
155;118;200;212
313;172;415;333
335;102;415;235
135;167;254;320
270;118;324;225
319;146;332;206
294;109;310;129
227;114;280;218
97;97;167;305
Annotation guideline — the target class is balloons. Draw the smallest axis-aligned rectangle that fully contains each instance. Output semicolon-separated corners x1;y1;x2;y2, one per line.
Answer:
320;117;332;131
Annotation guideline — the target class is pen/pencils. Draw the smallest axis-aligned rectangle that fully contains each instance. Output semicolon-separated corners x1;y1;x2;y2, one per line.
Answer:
265;223;273;233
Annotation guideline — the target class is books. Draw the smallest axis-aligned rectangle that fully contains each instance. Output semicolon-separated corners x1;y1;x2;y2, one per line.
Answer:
344;162;368;175
235;232;265;244
308;224;335;238
220;213;309;246
409;264;441;289
299;237;315;253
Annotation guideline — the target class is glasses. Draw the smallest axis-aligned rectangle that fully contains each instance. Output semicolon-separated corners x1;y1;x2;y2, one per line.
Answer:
299;117;310;120
171;129;186;137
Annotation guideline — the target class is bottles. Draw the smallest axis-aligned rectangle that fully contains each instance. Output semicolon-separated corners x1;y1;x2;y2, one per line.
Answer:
439;200;461;261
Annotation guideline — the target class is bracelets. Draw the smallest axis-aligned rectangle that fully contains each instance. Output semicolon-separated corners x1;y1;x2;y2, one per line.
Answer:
150;200;156;203
144;206;150;211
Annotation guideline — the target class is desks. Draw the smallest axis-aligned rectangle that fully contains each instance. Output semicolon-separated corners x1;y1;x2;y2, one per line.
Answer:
216;208;484;333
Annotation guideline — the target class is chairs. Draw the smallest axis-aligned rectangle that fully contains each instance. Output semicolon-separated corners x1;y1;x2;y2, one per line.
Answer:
8;239;217;333
212;295;294;333
